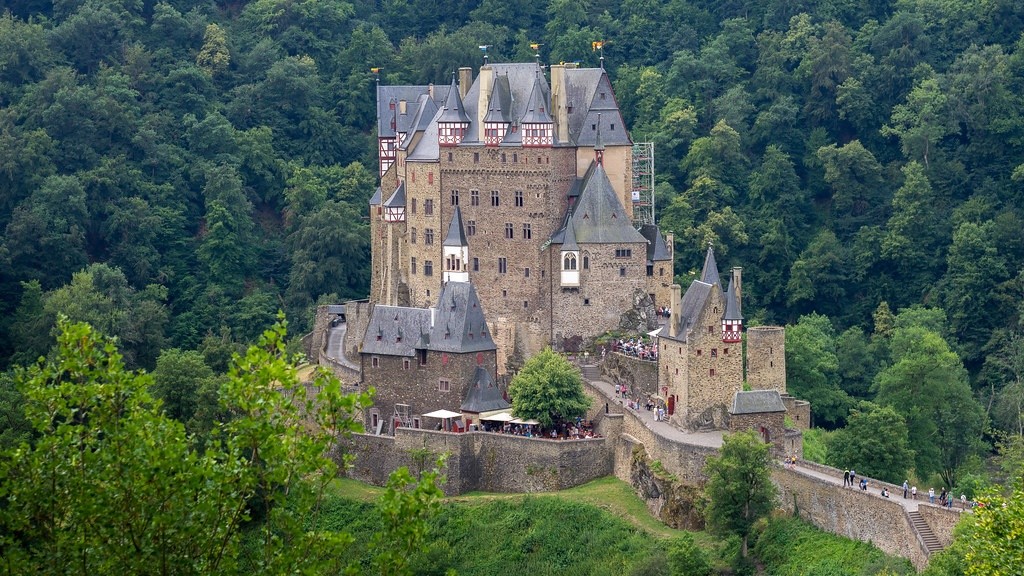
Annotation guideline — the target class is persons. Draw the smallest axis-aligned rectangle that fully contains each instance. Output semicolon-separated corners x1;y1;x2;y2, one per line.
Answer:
972;500;985;509
859;478;869;491
939;487;945;500
885;489;890;498
880;487;886;496
941;494;947;507
903;480;908;498
911;485;917;500
928;487;935;504
784;455;797;470
843;467;850;487
948;492;953;508
960;492;966;511
403;300;668;442
850;469;855;485
